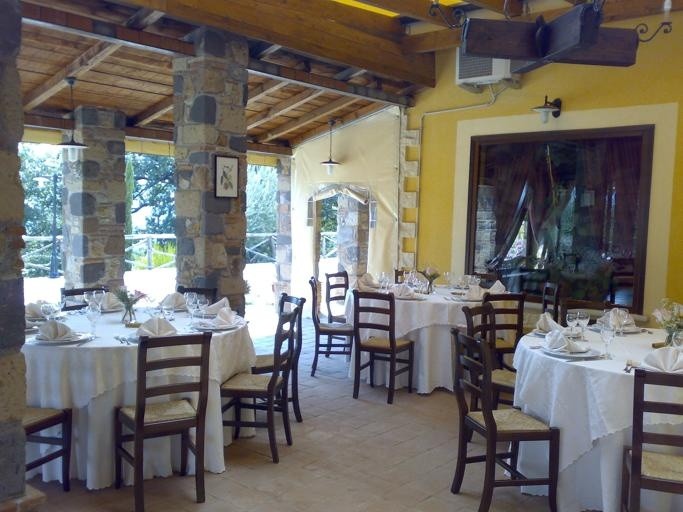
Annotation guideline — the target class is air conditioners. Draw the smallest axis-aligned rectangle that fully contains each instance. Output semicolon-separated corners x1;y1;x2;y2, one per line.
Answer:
454;43;522;89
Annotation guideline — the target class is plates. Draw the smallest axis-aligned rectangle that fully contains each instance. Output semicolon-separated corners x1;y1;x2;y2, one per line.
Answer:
534;328;548;336
195;319;238;331
589;327;642;333
458;296;485;302
395;293;427;300
34;332;94;344
189;306;229;318
542;344;606;358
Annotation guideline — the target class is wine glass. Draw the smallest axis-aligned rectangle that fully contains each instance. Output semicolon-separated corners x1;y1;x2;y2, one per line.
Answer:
564;312;580;342
184;292;196;305
186;299;197;325
198;299;209;319
600;324;617;360
674;330;683;348
378;272;423;291
577;313;592;341
83;288;107;337
443;272;482;292
42;294;66;323
615;309;631;337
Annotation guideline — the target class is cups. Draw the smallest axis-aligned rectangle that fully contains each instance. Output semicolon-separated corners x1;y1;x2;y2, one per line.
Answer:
163;303;174;322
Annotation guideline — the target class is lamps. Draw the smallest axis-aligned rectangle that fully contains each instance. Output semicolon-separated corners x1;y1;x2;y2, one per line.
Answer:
320;118;340;174
53;76;89;162
426;1;675;76
530;94;563;124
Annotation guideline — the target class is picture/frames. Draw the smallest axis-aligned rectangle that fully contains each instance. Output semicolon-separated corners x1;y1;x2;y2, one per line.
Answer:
214;155;239;199
